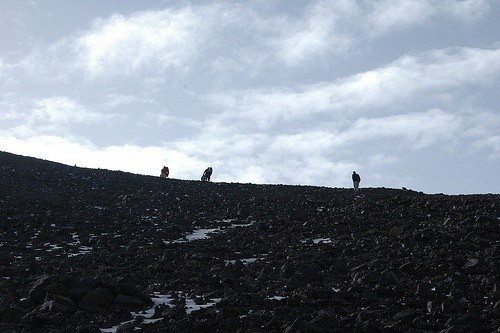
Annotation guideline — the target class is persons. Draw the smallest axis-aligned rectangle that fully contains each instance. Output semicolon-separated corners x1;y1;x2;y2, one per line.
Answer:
205;167;212;180
204;168;209;180
352;171;361;188
160;166;168;178
